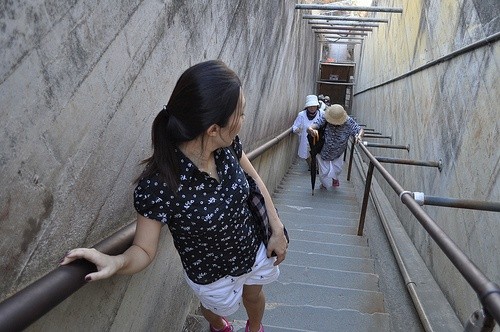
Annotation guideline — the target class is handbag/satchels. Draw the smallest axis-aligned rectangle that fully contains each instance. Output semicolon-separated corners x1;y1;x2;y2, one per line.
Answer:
243;169;289;257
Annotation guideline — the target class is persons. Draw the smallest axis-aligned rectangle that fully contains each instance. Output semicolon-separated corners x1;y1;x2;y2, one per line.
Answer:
318;93;331;112
306;103;364;191
62;60;290;332
291;94;325;175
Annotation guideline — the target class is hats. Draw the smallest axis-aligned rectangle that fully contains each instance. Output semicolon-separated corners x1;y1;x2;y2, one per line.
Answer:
304;95;321;110
325;104;348;125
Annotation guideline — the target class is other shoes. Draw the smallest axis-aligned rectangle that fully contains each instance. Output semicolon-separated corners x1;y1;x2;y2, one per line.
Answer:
209;316;235;332
333;179;339;186
245;319;264;332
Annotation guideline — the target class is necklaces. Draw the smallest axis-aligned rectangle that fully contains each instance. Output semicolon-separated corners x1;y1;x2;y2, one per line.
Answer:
196;156;214;175
